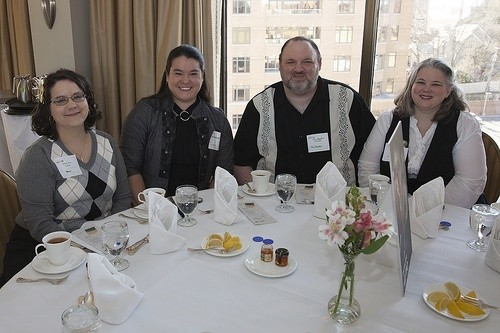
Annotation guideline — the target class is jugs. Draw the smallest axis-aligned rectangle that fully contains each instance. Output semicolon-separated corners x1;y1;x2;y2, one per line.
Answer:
13;73;33;103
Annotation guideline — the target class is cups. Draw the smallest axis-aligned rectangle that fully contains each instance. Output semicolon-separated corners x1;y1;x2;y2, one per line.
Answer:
251;170;270;194
60;303;101;333
34;231;71;265
138;187;167;211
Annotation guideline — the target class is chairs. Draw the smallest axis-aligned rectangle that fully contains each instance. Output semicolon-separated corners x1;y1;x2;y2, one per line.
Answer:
481;132;500;203
0;168;21;277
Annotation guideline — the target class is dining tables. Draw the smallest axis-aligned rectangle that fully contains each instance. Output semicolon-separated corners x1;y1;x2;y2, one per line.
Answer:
0;181;500;333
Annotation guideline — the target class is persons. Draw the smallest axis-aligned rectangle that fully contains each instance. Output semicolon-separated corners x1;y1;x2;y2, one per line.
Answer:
2;68;133;284
119;45;233;206
233;36;377;184
357;58;487;209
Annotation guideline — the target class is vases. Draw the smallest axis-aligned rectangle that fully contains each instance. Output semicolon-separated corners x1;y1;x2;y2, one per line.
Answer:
328;273;361;325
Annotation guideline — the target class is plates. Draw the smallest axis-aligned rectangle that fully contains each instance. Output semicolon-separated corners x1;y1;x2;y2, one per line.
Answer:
201;231;250;257
422;282;489;322
33;246;87;274
242;181;277;196
135;204;149;220
245;249;298;278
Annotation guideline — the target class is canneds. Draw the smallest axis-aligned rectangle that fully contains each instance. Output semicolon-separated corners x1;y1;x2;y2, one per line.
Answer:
260;244;274;262
275;248;289;267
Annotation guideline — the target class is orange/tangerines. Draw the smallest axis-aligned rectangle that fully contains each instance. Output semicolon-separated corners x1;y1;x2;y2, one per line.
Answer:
435;297;450;310
206;231;242;251
456;301;485;315
444;282;461;301
448;299;465;319
427;291;444;302
459;290;476;302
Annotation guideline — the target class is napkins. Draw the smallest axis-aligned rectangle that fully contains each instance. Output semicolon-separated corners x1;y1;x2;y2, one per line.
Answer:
88;253;143;325
144;192;186;255
313;160;347;220
483;212;500;274
408;176;446;241
212;166;246;226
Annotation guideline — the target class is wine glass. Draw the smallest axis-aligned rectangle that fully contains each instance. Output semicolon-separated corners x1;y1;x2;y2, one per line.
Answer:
175;184;199;227
467;203;500;253
275;173;296;213
367;173;390;210
100;220;129;272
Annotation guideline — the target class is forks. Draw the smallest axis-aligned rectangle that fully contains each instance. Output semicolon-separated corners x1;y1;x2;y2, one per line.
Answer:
460;293;500;311
16;274;69;285
195;206;214;214
118;213;148;224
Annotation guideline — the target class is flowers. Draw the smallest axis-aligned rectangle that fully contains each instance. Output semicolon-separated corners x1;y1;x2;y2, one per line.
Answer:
318;181;394;314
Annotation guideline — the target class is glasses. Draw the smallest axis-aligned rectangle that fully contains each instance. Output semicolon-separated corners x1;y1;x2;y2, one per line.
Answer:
49;92;87;106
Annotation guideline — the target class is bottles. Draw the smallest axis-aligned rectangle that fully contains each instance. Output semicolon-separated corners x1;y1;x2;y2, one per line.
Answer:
260;239;274;262
275;248;289;266
252;236;264;260
